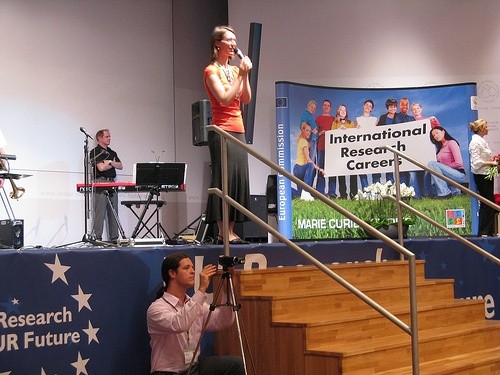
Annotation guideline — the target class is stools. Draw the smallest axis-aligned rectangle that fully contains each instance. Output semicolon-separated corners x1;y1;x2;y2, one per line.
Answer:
121;201;167;238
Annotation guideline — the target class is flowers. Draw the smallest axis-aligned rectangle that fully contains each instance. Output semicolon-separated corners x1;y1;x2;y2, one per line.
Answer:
484;153;500;181
353;180;416;226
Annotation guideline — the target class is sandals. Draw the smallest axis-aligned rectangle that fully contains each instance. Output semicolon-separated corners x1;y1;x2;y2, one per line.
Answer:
216;234;250;244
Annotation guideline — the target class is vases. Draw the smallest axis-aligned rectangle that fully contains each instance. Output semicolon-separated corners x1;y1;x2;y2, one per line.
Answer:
364;224;410;238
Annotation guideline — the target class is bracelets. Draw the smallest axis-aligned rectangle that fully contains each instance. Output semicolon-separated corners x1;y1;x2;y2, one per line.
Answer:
109;160;111;165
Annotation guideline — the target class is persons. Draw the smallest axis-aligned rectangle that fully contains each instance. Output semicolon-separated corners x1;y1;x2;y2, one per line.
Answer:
376;98;466;199
316;99;379;201
0;128;8;187
204;26;252;244
147;252;245;375
300;99;325;188
468;118;500;237
291;122;326;200
90;129;123;241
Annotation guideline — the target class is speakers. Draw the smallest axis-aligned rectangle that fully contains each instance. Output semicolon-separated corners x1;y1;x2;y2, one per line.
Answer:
0;220;23;249
192;99;211;145
244;195;268;237
266;175;277;213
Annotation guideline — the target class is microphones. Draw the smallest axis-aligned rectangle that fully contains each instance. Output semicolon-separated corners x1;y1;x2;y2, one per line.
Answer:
234;47;244;59
80;126;93;139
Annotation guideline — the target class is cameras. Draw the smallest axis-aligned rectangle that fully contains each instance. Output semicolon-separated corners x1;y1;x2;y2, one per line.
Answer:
218;255;245;271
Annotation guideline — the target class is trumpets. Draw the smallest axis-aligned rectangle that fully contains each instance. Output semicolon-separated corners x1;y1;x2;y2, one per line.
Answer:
0;159;25;199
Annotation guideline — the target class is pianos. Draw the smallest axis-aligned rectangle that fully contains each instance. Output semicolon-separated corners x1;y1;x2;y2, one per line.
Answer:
76;182;186;239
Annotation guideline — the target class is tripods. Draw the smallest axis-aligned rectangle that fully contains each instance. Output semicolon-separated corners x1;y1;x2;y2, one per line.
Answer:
186;270;256;375
55;136;113;248
